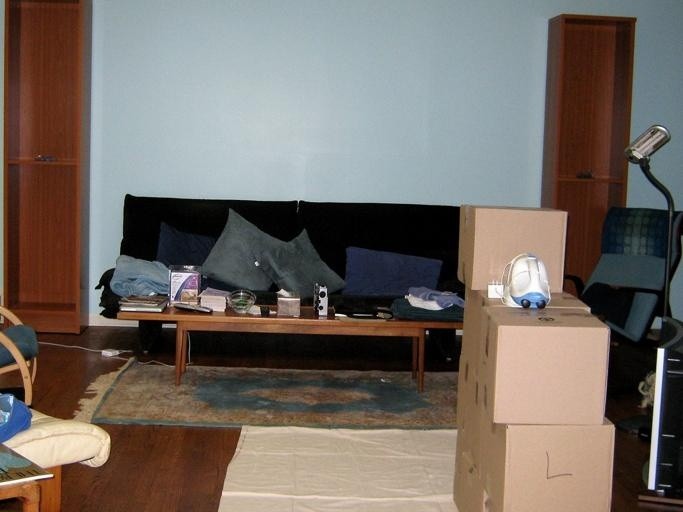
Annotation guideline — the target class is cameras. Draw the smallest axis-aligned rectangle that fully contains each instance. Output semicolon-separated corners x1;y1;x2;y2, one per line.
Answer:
313;282;328;317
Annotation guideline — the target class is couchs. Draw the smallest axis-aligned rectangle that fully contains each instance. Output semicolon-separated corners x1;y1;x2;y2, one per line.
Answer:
101;193;462;356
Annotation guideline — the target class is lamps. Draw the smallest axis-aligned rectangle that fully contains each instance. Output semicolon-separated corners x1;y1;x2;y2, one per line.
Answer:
624;125;683;504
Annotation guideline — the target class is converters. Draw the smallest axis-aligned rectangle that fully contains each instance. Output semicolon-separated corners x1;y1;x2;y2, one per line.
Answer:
101;348;119;357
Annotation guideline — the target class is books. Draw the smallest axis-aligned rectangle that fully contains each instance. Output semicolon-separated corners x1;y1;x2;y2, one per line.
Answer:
0;441;56;485
118;294;169;312
197;287;232;312
332;303;396;323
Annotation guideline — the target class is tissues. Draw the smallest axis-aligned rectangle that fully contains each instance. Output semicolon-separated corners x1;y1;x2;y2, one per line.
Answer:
277;288;302;317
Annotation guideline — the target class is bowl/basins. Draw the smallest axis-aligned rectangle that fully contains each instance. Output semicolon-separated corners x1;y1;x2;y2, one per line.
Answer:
227;290;257;314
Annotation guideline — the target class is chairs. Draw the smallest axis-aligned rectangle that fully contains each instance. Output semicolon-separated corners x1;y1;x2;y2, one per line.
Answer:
1;307;62;512
561;206;682;382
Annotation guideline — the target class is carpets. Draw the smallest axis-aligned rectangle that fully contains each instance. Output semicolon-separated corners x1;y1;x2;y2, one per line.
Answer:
67;358;457;430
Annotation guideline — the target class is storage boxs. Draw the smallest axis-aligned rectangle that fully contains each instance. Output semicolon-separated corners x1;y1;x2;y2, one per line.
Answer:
452;205;617;512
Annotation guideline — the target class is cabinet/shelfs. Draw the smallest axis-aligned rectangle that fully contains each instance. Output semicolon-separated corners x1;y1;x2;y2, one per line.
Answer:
541;14;636;298
3;0;86;335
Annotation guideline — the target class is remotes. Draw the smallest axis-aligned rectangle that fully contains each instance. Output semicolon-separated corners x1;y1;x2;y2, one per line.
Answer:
173;302;213;313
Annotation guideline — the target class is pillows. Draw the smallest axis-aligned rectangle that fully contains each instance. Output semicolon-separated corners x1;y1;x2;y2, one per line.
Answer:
197;208;441;300
